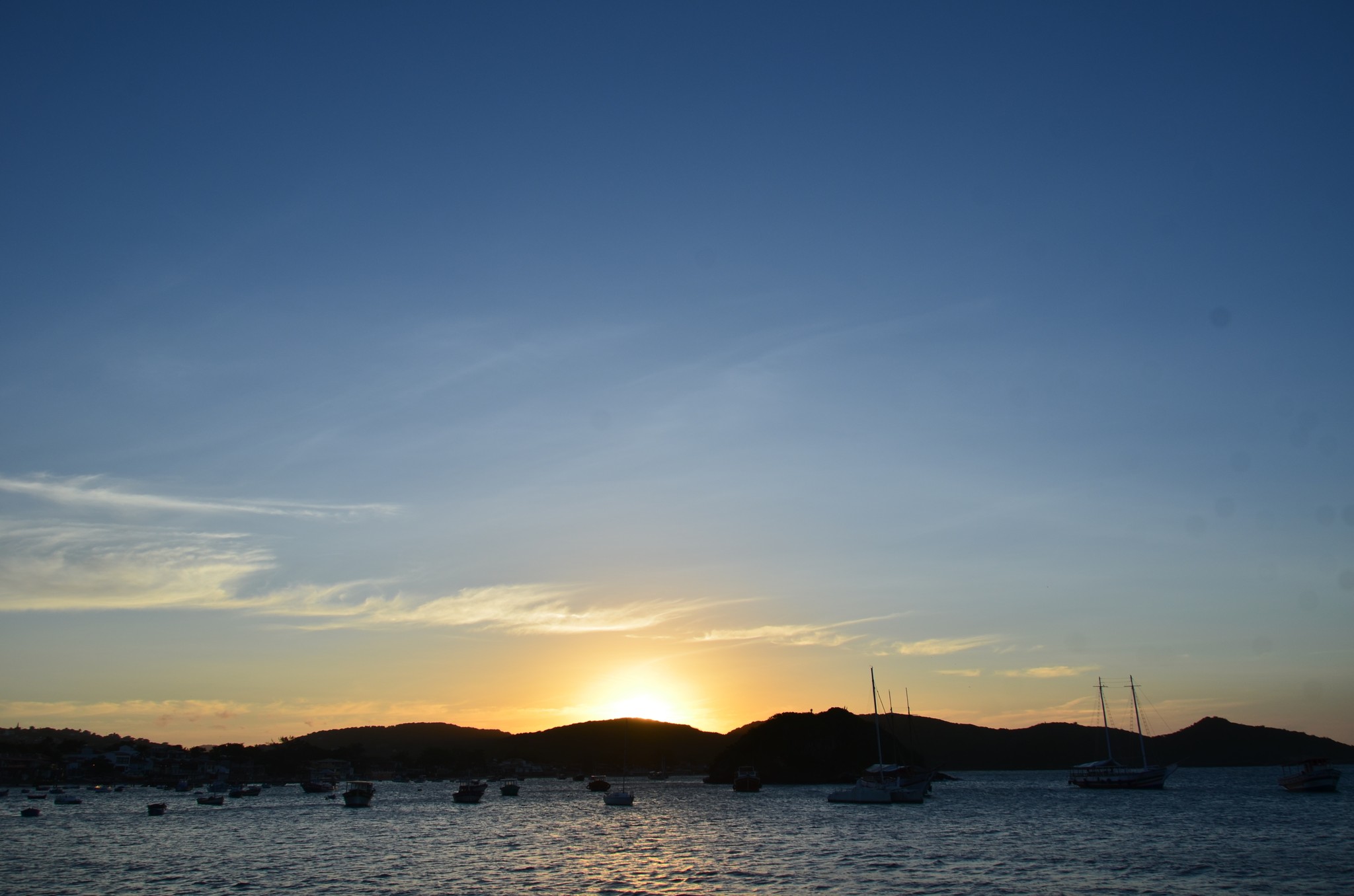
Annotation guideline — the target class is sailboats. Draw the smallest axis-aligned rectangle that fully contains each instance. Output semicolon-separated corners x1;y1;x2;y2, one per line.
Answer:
823;665;1179;806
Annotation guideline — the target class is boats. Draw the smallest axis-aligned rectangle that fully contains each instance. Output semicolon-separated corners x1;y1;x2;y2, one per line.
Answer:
498;778;520;795
265;773;376;806
231;784;262;796
147;802;167;812
453;779;489;804
21;789;30;793
1277;763;1343;792
21;808;41;817
392;766;867;807
36;785;80;794
0;789;10;796
27;794;47;799
87;783;130;793
194;791;203;794
196;795;224;806
156;780;193;793
55;795;83;805
262;783;272;788
206;779;230;792
